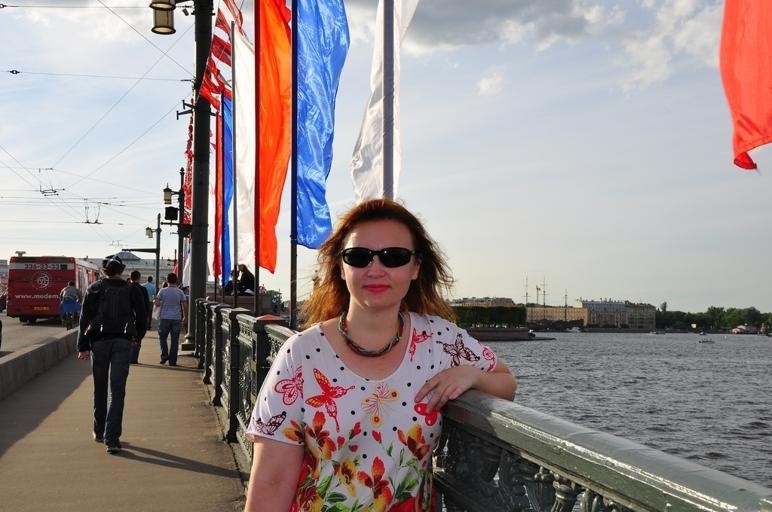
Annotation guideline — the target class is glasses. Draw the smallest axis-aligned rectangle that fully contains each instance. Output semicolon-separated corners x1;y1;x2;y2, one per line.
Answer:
336;247;419;269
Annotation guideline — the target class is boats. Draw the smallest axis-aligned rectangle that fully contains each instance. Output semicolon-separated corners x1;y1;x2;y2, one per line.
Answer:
469;323;535;341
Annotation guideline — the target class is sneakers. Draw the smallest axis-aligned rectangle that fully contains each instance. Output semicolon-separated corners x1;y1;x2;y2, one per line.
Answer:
92;429;121;453
159;355;178;366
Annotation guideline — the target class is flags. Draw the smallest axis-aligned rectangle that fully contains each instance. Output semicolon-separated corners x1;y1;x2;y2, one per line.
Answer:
716;0;772;174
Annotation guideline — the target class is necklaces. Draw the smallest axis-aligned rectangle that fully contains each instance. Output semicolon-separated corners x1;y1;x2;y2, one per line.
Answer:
336;309;406;358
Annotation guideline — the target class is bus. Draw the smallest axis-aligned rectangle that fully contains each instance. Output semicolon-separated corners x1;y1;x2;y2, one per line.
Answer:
6;251;98;323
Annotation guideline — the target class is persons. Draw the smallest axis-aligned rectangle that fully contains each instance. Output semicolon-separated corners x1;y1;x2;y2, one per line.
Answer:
155;273;188;366
59;264;254;331
76;254;147;453
242;198;519;512
129;270;150;364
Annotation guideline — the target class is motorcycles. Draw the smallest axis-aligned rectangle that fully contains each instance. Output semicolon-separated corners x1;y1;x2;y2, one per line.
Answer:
61;296;80;330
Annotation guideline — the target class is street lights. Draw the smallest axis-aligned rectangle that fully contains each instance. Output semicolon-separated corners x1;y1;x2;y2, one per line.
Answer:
149;0;215;344
146;213;161;296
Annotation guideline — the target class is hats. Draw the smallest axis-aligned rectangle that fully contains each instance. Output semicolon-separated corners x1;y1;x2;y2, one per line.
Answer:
101;254;125;268
